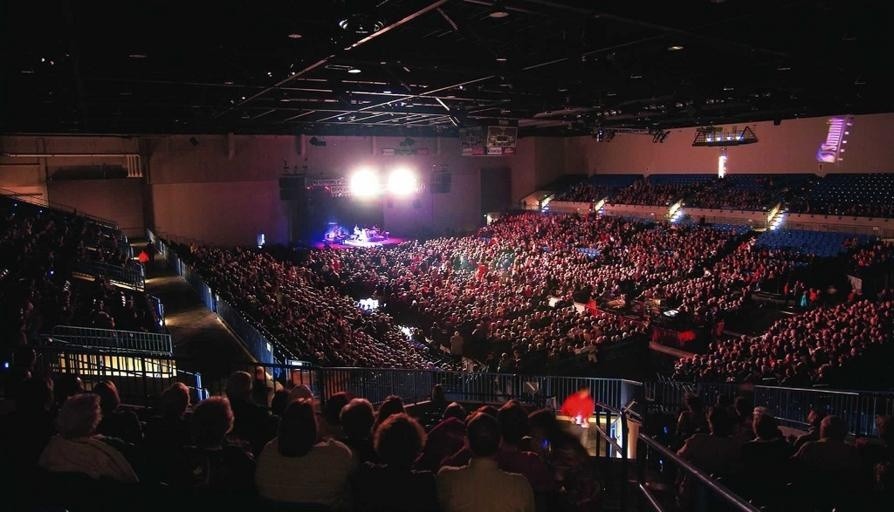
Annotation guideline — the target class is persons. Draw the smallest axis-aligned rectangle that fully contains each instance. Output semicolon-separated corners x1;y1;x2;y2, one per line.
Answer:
0;176;894;512
